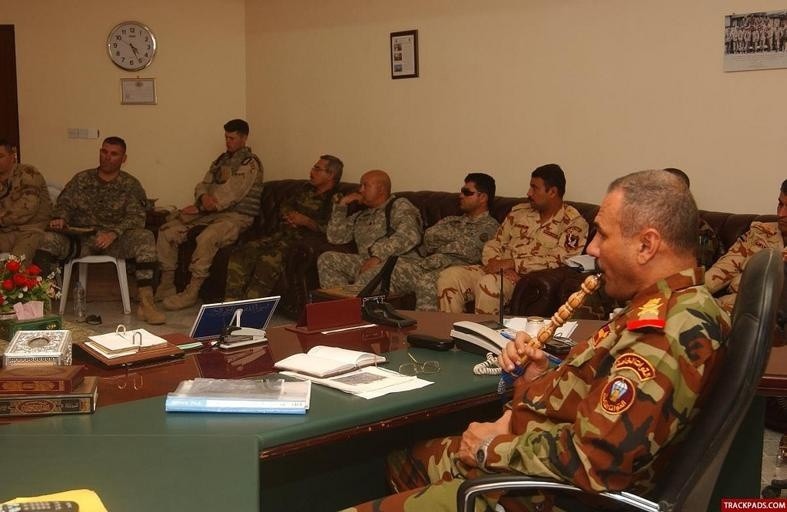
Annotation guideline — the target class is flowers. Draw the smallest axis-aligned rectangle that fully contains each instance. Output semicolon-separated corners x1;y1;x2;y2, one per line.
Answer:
0;248;68;317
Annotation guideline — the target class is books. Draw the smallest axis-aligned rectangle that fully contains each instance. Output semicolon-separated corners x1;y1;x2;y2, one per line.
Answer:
84;325;181;359
0;364;86;392
165;377;311;415
273;346;389;378
0;376;98;417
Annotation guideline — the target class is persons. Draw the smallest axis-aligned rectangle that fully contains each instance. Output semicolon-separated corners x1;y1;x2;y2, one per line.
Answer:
568;168;726;319
374;173;503;311
33;136;167;323
318;169;427;296
304;170;732;512
725;14;787;53
704;178;786;318
437;164;590;315
155;119;263;310
225;154;345;302
0;139;53;270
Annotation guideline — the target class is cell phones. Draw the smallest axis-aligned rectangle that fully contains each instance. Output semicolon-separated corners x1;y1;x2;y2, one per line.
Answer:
407;334;454;352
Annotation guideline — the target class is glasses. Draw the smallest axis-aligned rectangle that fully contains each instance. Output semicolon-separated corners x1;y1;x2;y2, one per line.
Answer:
399;353;440;376
461;186;481;197
77;315;103;325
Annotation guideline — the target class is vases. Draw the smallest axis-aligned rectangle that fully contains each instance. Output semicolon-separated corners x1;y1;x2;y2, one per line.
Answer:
1;297;51;324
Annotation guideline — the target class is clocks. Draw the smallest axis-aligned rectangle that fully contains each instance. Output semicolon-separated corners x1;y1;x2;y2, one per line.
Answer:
104;20;160;73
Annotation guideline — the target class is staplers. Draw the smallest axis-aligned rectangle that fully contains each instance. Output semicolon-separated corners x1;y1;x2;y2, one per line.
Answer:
220;327;267;350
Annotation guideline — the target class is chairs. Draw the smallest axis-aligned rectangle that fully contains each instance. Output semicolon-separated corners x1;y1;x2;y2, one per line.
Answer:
450;245;785;512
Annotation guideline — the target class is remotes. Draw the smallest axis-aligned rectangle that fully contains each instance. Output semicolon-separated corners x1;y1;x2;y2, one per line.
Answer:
3;501;78;512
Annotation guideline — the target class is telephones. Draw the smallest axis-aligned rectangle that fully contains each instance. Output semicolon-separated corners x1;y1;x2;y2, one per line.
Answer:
451;319;519;357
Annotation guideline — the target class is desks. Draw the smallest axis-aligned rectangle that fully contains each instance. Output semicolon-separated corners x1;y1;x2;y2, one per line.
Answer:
0;305;786;511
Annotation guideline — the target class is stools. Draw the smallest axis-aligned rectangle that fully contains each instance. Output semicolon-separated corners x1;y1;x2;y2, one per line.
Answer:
54;250;134;322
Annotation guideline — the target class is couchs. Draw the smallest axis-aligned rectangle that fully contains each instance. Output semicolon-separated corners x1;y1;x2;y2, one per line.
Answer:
161;173;782;344
31;197;177;306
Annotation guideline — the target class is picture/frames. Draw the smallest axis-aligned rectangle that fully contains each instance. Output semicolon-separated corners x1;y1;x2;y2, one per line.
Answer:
387;28;422;83
118;73;158;107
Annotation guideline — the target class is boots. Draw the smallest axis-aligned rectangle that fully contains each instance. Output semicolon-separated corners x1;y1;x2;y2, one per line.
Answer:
136;271;206;324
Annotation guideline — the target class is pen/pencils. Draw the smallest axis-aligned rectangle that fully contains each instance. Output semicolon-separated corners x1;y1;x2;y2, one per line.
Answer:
501;330;562;364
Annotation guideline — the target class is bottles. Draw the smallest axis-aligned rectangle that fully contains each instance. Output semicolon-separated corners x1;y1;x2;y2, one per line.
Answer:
524;315;546;337
71;281;86;323
